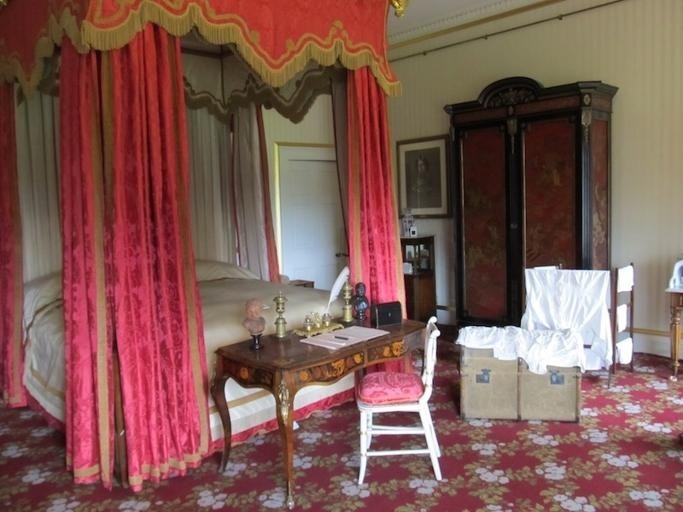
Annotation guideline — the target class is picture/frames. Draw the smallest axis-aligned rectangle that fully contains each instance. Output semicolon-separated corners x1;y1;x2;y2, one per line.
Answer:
396;134;452;220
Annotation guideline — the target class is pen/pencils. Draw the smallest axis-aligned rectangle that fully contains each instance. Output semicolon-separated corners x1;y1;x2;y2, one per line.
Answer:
334;336;348;340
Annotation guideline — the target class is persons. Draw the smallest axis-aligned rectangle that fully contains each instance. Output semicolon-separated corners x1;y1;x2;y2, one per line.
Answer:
350;282;369;312
407;159;434;194
241;297;265;334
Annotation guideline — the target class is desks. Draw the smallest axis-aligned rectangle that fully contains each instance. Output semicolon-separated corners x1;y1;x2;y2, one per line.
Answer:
210;316;426;510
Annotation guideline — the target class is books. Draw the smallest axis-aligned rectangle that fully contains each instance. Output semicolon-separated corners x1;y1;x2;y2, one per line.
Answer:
299;325;391;352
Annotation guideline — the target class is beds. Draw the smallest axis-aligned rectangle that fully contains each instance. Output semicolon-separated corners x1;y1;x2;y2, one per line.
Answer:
22;261;360;487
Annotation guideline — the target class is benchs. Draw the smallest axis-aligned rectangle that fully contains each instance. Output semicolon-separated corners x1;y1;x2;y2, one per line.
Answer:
459;326;582;422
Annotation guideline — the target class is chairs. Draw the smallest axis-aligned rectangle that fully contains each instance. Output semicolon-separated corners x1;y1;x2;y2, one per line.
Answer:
356;316;443;485
